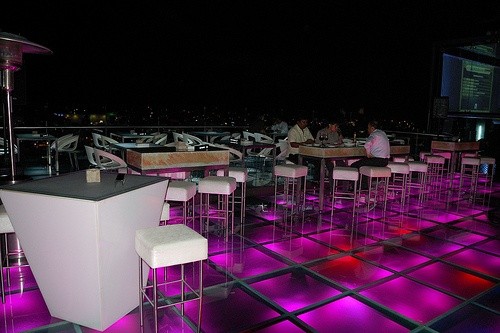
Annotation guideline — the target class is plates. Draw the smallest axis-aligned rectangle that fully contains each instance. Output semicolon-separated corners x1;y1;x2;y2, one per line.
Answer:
241;140;253;144
303;142;344;147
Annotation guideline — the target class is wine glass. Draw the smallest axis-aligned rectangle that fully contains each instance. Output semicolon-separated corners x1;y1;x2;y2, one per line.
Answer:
319;132;327;147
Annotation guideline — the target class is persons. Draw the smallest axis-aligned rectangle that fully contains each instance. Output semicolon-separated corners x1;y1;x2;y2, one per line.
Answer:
315;116;354;201
288;118;321;186
0;106;366;146
350;122;390;209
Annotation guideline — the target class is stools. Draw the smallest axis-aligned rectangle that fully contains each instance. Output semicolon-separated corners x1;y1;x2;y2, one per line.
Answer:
331;167;359;219
160;167;248;244
459;151;496;191
134;224;208;333
274;164;307;218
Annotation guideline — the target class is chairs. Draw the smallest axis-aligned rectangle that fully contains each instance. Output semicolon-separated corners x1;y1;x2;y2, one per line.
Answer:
358;152;452;212
85;132;127;173
139;131;288;166
51;133;80;171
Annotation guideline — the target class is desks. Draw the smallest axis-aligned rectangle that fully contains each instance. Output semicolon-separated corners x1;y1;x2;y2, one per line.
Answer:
0;170;170;331
111;143;167;159
296;145;410;213
15;133;59;171
213;139;276;183
430;141;479;173
126;148;229;238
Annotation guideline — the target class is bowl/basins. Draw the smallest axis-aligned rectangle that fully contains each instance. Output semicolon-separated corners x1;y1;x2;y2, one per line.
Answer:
344;142;356;147
